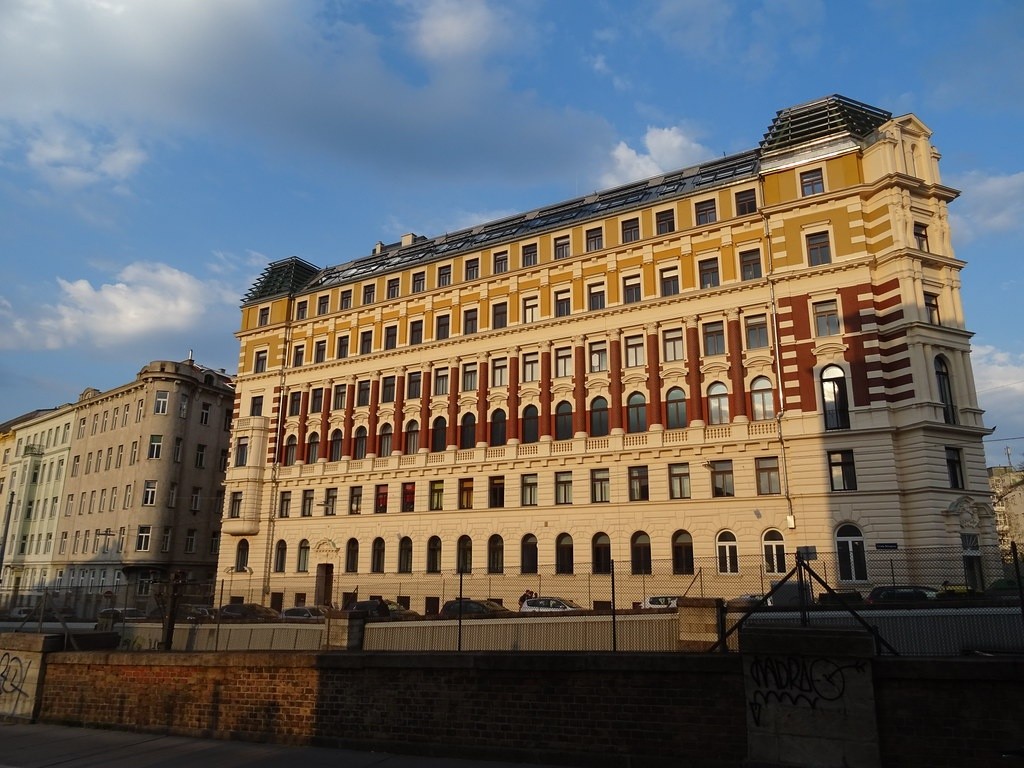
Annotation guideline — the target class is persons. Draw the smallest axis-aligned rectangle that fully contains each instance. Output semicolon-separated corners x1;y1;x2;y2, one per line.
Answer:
534;593;537;598
518;590;534;612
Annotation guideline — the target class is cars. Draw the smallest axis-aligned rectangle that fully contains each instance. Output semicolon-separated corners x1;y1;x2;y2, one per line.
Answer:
47;607;76;622
10;606;35;621
520;597;584;611
216;604;279;623
0;609;9;620
724;594;771;606
439;597;512;615
96;607;147;622
278;606;325;623
198;608;218;618
354;600;421;621
147;604;210;623
866;586;958;606
639;596;678;609
